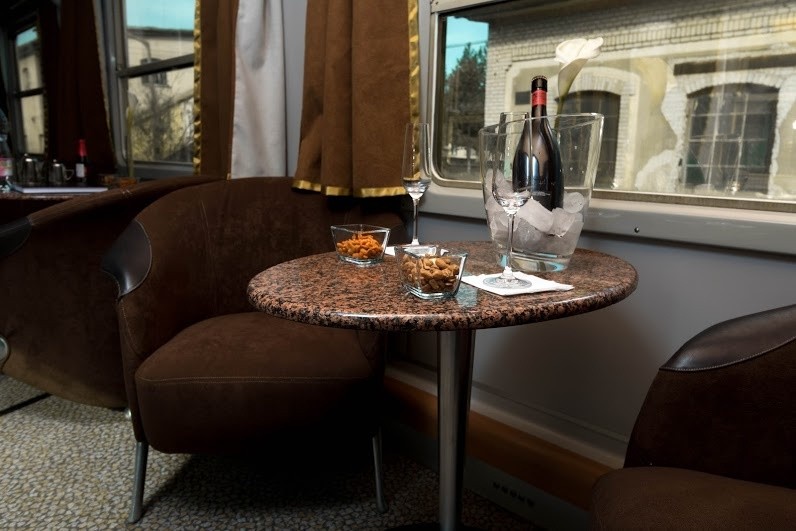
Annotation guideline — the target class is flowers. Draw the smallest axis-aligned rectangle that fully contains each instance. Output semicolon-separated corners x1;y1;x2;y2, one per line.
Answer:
553;35;603;138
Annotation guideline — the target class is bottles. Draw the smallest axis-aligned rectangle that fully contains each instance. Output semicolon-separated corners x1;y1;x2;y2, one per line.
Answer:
512;75;564;239
73;138;91;188
0;135;14;193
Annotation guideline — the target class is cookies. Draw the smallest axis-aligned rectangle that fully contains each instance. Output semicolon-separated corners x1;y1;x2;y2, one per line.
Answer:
337;235;383;259
401;253;459;292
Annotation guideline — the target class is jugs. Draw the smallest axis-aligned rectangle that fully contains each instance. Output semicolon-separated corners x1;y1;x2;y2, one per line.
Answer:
16;154;74;187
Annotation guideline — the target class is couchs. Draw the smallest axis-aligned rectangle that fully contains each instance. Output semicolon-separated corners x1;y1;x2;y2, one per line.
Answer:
0;179;391;522
582;304;796;531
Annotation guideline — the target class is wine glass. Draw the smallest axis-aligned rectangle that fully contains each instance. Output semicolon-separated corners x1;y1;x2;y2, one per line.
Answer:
491;111;534;289
400;120;439;252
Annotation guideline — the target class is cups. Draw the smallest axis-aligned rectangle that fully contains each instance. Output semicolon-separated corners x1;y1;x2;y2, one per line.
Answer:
330;223;392;266
478;110;605;273
104;173;139;191
393;244;470;299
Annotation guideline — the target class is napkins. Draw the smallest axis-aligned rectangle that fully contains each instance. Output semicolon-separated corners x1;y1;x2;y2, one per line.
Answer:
384;245;449;258
461;271;575;296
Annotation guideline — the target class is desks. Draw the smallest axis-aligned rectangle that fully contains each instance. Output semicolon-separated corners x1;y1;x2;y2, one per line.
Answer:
245;241;638;531
0;190;94;210
